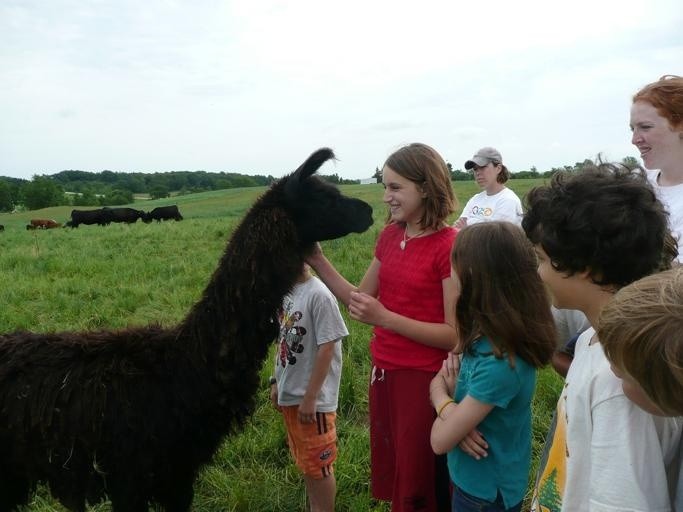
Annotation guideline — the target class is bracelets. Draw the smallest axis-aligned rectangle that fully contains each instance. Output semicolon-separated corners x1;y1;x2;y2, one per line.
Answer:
435;398;456;419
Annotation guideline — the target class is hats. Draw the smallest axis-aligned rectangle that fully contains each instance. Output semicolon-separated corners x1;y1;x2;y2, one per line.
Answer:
465;147;502;170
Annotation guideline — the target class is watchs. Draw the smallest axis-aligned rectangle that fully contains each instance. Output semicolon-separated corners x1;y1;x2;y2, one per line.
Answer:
267;375;276;385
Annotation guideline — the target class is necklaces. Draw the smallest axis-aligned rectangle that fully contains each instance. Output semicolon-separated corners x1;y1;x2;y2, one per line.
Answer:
398;220;445;251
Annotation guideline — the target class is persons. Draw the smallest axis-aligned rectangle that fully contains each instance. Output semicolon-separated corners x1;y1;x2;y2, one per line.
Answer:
542;301;593;379
427;221;557;511
447;146;528;233
268;261;349;511
303;142;461;510
594;265;683;416
626;74;682;271
516;155;683;512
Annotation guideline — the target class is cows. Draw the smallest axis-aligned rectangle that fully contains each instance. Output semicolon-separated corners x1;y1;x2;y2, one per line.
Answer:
26;205;184;230
